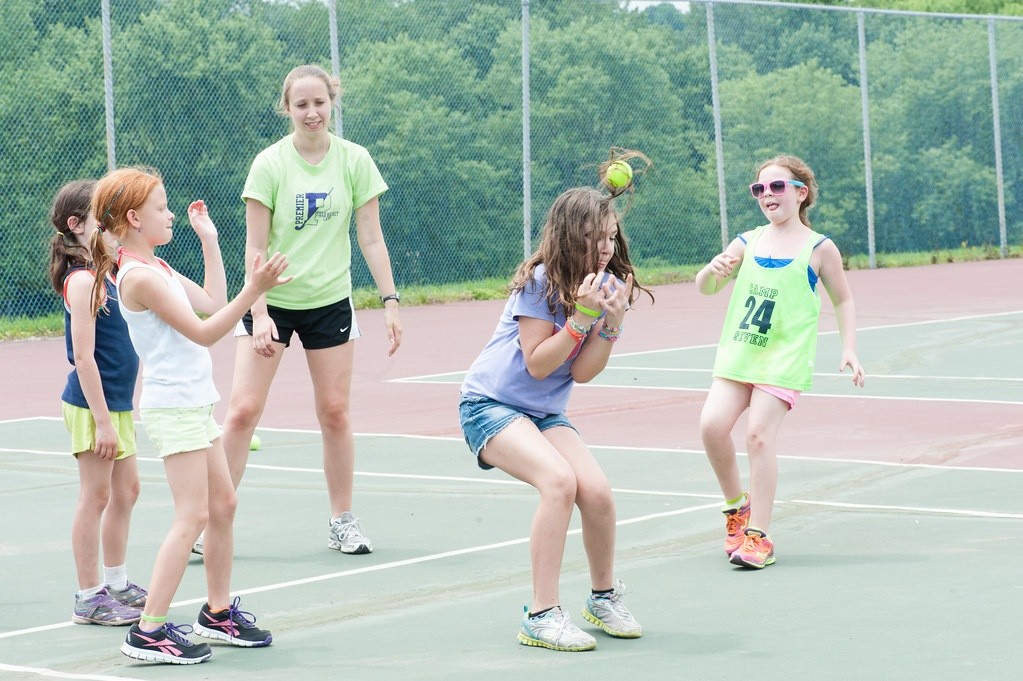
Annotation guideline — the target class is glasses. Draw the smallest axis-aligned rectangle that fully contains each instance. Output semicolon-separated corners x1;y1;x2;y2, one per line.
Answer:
747;179;806;199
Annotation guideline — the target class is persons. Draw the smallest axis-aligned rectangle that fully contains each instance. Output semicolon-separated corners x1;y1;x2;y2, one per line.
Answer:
52;180;149;626
192;65;404;555
90;167;293;665
695;155;866;569
459;187;644;652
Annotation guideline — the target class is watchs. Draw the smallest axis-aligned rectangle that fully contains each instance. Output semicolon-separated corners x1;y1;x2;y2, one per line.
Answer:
379;292;400;305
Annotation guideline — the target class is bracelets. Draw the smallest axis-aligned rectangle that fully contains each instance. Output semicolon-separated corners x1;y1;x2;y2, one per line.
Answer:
597;322;624;341
565;316;591;341
575;303;602;317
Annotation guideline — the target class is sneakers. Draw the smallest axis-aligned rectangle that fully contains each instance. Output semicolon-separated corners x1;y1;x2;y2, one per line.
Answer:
518;606;597;652
73;583;149;625
723;493;779;570
193;602;273;651
329;513;372;555
121;623;213;666
584;591;644;638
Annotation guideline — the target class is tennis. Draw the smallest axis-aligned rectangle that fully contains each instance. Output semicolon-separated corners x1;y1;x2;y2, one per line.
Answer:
605;160;633;188
249;435;260;450
